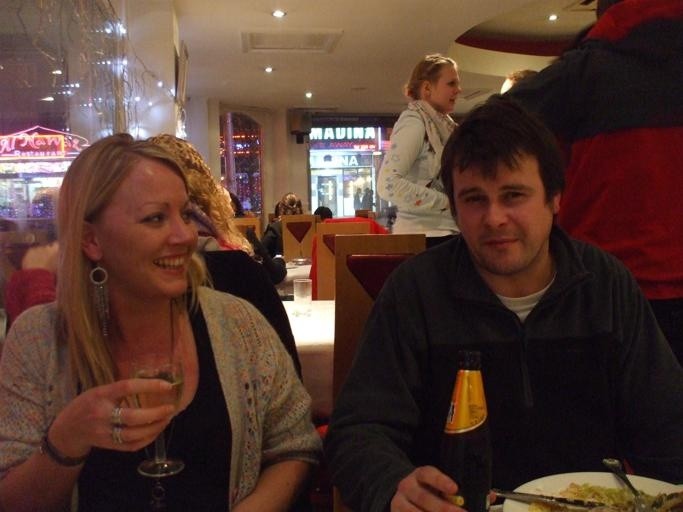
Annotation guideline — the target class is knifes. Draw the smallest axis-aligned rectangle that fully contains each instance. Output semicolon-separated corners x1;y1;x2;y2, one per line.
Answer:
489;487;607;509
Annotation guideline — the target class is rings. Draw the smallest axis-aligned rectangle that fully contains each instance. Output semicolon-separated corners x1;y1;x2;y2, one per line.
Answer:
111;406;124;425
112;426;123;444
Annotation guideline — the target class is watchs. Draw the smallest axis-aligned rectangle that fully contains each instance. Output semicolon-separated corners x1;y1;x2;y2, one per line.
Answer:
39;415;91;467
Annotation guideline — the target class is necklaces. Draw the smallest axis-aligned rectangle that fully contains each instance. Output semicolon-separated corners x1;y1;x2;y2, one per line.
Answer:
124;299;173;512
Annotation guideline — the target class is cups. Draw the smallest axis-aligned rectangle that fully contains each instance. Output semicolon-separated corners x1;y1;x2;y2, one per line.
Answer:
294;279;313;317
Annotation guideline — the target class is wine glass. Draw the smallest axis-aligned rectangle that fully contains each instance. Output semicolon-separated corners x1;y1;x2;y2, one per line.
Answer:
130;346;186;478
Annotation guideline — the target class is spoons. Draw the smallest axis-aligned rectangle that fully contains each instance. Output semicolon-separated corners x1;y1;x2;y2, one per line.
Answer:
604;457;659;510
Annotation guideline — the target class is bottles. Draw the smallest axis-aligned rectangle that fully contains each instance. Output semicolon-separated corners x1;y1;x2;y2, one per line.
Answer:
445;350;495;508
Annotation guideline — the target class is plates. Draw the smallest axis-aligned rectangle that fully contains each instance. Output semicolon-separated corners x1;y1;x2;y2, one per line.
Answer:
503;469;681;512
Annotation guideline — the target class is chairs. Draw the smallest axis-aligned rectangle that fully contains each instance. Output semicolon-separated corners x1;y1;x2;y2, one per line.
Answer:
215;212;425;512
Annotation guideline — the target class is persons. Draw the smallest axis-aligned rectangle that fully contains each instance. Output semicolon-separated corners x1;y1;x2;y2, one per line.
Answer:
376;55;460;237
230;192;287;283
262;192;303;259
322;93;682;511
147;134;303;383
314;206;332;222
2;240;62;324
1;132;322;512
500;1;683;364
